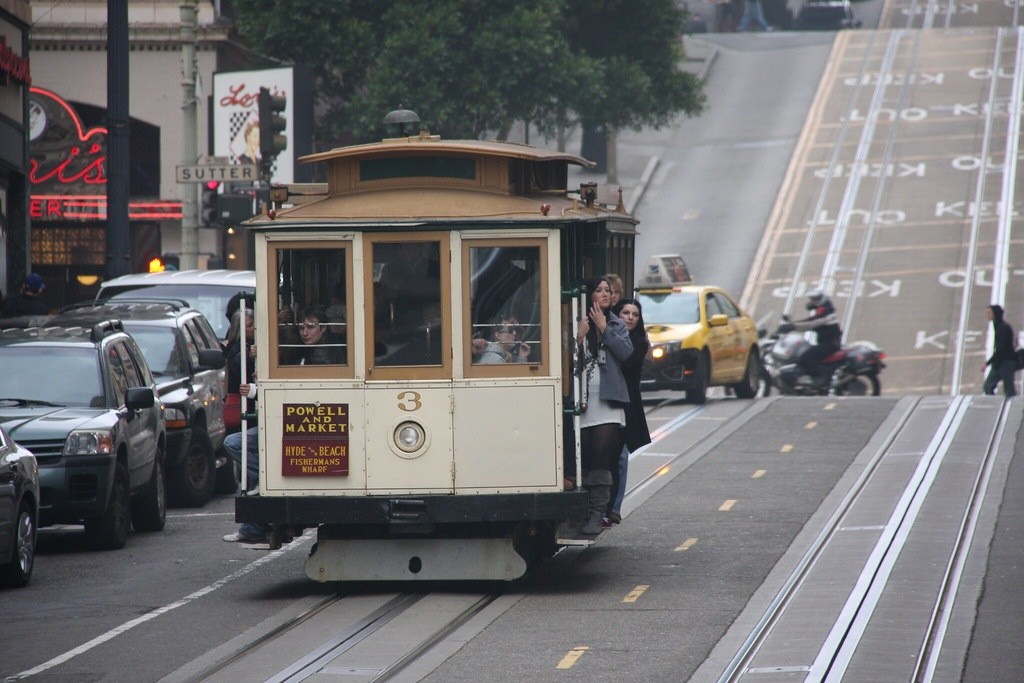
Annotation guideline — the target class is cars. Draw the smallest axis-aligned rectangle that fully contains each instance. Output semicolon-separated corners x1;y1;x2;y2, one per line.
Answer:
0;426;41;593
633;253;761;405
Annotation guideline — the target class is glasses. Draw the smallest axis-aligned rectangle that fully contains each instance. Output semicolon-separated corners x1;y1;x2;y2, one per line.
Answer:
499;327;514;333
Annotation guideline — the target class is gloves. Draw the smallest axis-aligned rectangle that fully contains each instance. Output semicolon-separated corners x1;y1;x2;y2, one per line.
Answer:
779;322;795;334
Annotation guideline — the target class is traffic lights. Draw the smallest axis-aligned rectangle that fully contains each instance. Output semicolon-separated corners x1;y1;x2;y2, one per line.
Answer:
257;86;287;153
202;178;222;226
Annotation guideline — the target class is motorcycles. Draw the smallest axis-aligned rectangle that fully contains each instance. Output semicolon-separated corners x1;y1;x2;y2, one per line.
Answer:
754;312;887;398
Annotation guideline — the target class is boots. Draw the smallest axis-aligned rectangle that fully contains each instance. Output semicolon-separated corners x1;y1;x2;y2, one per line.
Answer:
582;469;612;535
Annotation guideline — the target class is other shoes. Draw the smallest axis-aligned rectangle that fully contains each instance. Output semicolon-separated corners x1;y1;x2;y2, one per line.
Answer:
222;530;252;544
565;479;574;489
609;509;621;524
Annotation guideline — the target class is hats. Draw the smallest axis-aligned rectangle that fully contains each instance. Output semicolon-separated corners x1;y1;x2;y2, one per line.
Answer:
808;290;828;310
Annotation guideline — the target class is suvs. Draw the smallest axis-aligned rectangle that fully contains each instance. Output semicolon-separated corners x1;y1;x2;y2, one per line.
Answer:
792;0;862;30
0;320;169;550
51;297;232;508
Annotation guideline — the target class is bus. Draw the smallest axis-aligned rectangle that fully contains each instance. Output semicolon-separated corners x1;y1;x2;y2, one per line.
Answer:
234;107;640;594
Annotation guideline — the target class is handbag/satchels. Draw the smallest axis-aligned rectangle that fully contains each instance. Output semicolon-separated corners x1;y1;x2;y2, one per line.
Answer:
224;393;242;427
1011;346;1024;371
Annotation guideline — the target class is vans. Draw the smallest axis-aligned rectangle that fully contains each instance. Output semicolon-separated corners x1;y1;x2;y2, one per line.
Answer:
91;268;291;350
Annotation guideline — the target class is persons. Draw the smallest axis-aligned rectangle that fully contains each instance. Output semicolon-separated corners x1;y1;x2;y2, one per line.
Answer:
981;305;1017;397
0;273;48;329
297;307;346;364
223;292;294;543
781;290;843;392
472;314;531;363
565;273;652;533
